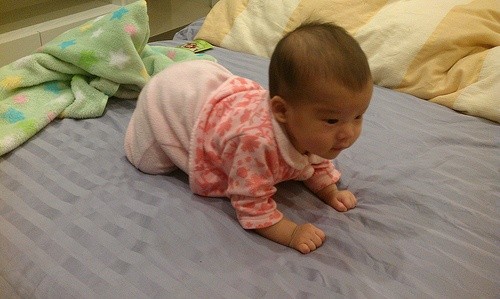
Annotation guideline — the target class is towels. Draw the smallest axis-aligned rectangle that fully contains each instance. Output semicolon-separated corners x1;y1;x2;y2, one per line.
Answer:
0;0;220;167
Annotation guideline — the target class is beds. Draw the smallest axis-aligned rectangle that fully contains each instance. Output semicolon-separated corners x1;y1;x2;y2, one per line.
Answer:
0;0;499;299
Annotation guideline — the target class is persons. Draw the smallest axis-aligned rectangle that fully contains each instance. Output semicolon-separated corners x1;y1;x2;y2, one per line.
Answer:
125;15;373;254
